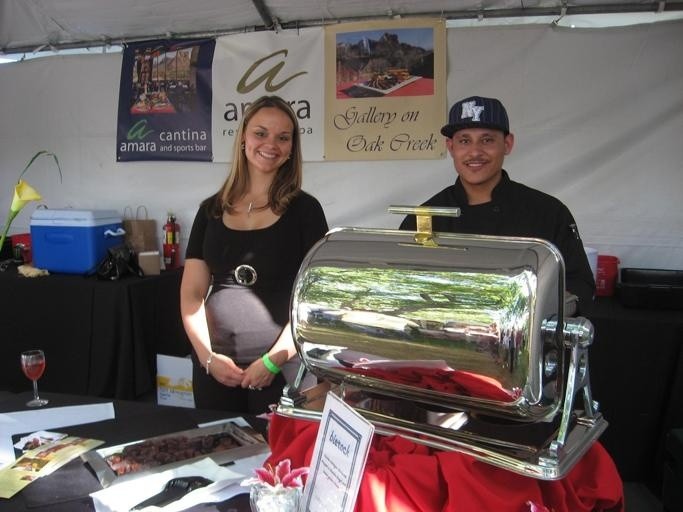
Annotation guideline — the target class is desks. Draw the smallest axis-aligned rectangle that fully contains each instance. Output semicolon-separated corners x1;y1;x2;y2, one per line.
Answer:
1;271;181;396
1;390;274;511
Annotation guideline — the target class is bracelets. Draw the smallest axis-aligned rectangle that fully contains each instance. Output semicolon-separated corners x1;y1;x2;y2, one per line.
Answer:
204;352;213;375
262;353;279;375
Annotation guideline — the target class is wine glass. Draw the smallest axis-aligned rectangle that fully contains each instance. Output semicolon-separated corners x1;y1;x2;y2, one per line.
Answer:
20;350;49;408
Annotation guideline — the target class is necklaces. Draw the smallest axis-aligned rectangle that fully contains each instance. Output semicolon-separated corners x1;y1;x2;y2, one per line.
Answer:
238;197;270;217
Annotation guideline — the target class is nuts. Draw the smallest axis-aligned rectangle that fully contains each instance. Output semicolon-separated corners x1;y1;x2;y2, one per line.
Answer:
111;432;239;476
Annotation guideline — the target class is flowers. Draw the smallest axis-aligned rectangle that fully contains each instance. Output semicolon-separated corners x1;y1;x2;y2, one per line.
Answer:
0;149;65;260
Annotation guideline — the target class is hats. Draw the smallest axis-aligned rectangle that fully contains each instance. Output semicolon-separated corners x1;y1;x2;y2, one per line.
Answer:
440;96;509;138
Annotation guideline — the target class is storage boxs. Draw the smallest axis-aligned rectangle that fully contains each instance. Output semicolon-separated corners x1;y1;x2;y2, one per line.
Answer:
30;207;124;276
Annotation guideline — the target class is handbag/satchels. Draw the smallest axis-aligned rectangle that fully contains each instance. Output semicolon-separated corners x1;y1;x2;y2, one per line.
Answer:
96;244;145;280
122;206;157;254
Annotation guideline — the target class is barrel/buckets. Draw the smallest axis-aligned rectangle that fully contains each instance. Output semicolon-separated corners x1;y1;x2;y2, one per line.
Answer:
597;254;621;296
12;233;32;262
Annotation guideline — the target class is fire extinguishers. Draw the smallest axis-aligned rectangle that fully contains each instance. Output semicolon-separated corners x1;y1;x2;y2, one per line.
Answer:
162;210;181;271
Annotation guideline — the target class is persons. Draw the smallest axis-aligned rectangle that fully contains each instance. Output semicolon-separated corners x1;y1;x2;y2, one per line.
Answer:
396;97;608;366
179;96;329;411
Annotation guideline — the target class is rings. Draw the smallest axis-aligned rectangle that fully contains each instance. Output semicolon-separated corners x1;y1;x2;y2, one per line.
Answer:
248;384;254;391
